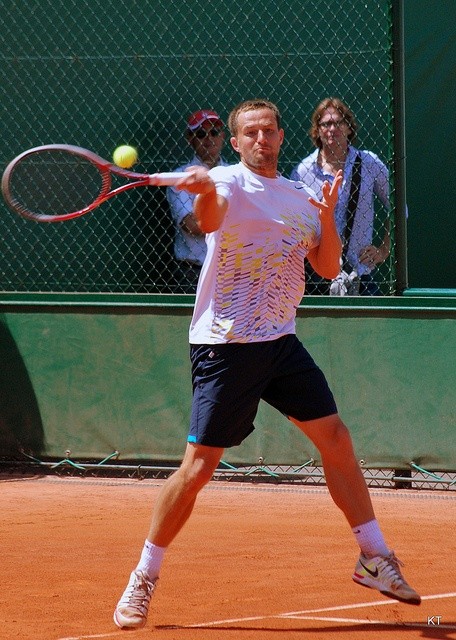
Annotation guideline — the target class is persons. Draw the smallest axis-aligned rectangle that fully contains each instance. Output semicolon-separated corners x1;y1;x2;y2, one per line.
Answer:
113;99;422;631
289;98;409;295
165;109;234;294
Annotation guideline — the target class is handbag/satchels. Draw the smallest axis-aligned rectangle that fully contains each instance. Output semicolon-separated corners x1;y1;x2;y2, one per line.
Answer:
304;258;360;296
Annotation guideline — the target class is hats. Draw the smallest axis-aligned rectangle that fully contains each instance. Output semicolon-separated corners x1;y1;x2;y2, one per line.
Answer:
186;110;224;129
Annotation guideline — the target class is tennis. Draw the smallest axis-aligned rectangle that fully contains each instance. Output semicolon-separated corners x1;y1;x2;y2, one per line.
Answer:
112;145;137;168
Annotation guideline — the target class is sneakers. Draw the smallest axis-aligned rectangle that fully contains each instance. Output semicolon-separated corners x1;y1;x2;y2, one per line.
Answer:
352;551;421;605
113;572;160;630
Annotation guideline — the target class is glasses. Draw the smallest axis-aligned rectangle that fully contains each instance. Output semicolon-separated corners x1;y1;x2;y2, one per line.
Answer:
190;129;223;140
319;119;346;127
